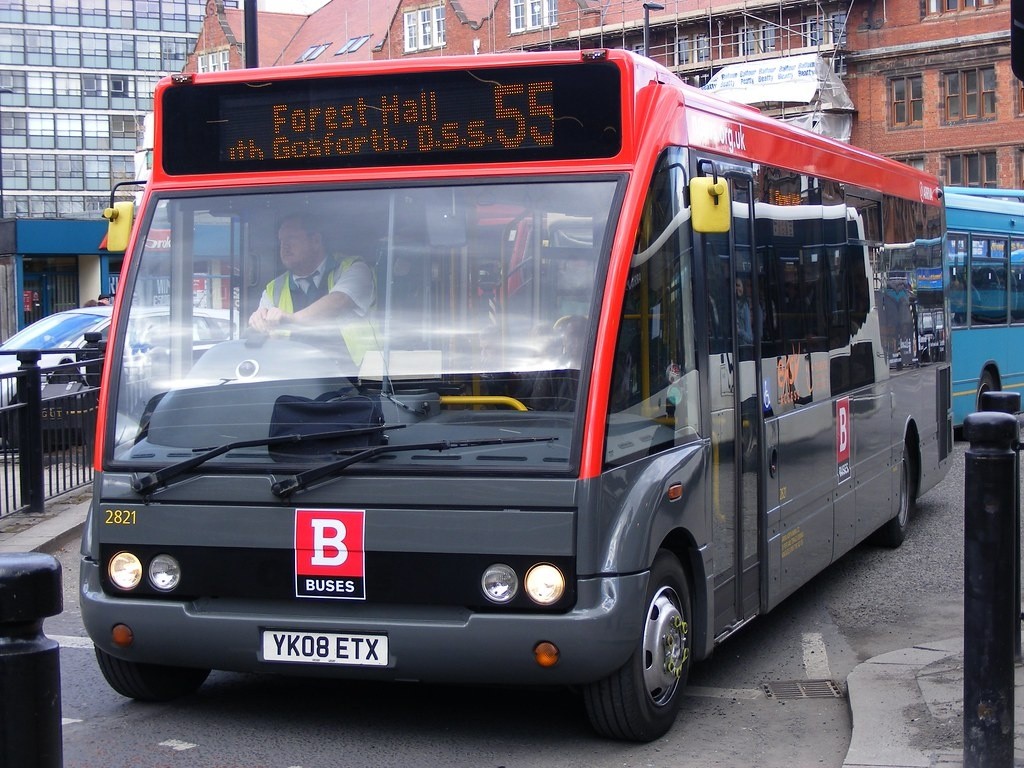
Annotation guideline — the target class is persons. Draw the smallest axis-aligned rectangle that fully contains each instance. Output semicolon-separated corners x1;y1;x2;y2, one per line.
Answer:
249;213;384;386
83;300;97;307
392;315;586;414
951;266;977;326
98;294;111;306
707;243;868;356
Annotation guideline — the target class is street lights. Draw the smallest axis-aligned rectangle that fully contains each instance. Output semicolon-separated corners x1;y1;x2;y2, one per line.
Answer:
643;1;665;59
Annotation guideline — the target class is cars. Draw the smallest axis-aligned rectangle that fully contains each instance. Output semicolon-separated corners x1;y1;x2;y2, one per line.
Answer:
1;308;240;408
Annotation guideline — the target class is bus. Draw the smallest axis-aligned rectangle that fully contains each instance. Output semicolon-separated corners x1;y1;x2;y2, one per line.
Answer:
79;46;952;743
881;235;1024;328
940;186;1023;443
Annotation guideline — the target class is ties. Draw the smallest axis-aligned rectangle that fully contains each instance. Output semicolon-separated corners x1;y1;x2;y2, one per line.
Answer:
298;270;321;300
